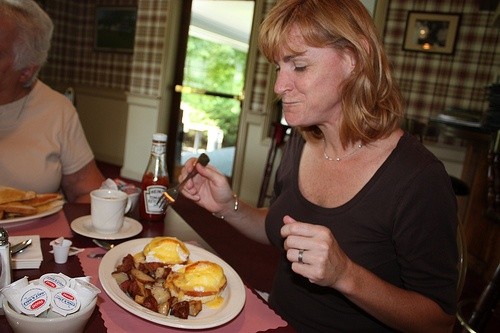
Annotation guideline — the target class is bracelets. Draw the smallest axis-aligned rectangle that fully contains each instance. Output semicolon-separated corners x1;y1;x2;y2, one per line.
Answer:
211;193;239;219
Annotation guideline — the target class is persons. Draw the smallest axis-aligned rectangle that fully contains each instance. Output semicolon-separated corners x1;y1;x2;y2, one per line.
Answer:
1;1;105;203
178;0;463;332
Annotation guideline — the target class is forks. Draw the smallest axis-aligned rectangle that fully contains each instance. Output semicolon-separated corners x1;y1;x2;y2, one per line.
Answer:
155;154;210;213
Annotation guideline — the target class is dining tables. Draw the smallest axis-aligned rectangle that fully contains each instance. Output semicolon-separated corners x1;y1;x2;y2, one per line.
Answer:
0;191;295;333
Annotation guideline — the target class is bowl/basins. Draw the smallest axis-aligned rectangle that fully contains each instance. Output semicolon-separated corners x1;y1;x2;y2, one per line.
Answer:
2;279;98;333
100;188;141;213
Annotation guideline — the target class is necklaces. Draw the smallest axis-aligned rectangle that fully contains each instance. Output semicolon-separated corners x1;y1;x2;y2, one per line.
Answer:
319;139;367;161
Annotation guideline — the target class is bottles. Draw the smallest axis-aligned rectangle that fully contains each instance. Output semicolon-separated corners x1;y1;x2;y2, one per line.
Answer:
140;133;170;221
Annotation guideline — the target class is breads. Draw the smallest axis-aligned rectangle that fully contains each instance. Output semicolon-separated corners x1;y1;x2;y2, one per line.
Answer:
0;186;65;219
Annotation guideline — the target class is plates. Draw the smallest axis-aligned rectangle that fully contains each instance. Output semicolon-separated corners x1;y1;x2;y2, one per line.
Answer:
0;199;63;224
98;238;246;329
70;215;143;239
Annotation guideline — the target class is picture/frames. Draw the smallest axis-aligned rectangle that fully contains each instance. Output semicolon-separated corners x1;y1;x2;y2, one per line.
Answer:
402;10;462;54
92;5;138;53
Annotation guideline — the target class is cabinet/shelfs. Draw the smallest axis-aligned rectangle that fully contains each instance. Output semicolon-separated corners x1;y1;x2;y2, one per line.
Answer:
419;134;486;273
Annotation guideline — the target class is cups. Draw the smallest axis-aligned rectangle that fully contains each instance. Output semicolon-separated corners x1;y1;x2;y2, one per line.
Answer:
90;189;132;234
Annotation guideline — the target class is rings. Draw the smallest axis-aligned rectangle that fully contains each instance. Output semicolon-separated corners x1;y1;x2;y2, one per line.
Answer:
297;249;306;264
